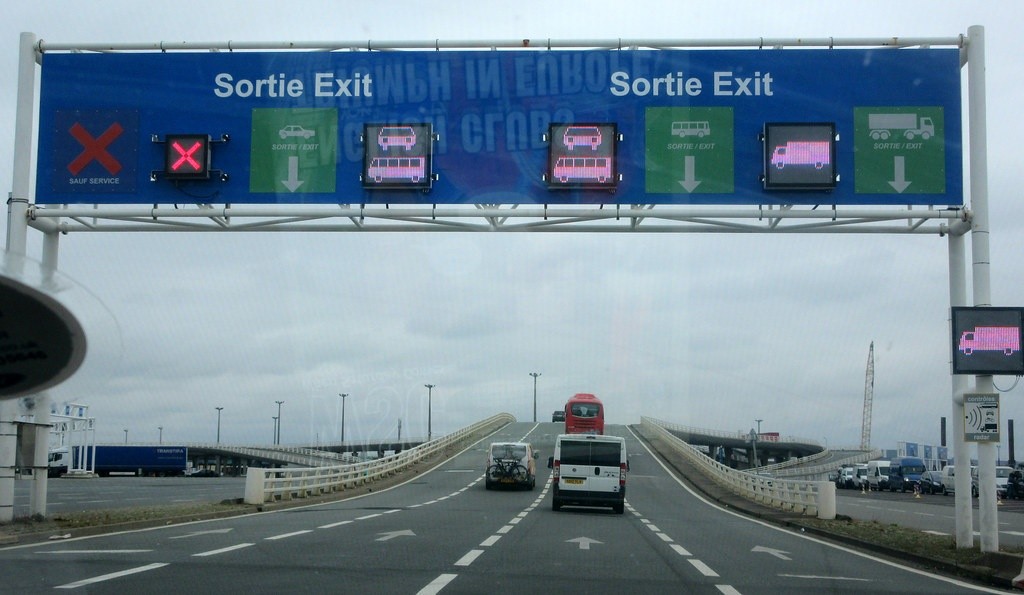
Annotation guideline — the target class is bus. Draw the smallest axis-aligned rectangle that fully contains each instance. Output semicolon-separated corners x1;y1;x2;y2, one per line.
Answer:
564;393;605;436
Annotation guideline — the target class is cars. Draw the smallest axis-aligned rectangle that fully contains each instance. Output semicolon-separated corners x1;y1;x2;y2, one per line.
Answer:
920;470;942;494
187;469;219;477
552;411;565;422
1008;470;1024;501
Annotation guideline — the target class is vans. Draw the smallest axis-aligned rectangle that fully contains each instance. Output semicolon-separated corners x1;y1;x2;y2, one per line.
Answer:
868;460;890;491
941;465;958;494
546;433;630;514
840;467;853;490
853;463;867;490
889;456;927;492
486;442;540;491
971;466;1016;499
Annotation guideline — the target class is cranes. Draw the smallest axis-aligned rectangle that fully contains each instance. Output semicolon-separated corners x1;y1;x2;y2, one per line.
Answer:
860;340;876;451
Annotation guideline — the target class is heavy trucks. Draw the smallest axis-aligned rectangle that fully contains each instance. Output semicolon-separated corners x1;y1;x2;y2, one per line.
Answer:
47;445;188;478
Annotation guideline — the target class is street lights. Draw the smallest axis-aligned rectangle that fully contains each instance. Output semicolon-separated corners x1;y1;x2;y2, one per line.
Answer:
756;419;763;435
272;417;278;444
425;384;436;442
338;393;350;442
124;430;128;443
158;427;162;444
530;372;542;422
215;407;223;442
275;401;285;444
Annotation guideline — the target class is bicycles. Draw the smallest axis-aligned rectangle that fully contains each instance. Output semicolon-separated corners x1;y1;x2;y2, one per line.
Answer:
487;457;529;484
